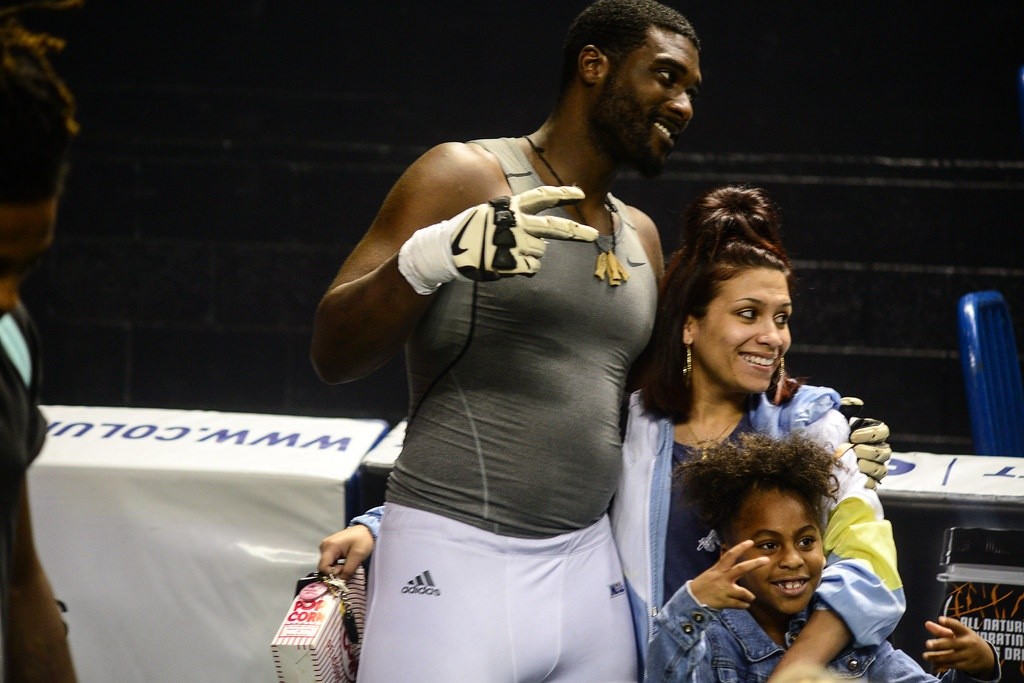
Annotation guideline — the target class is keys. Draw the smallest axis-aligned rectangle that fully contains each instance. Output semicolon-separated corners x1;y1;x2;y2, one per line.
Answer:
322;571;360;640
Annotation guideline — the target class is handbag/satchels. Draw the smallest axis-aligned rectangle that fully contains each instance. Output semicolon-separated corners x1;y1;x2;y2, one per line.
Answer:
270;558;367;683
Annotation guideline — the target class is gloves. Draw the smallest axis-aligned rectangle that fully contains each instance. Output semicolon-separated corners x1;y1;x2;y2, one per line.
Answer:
451;186;599;284
837;397;892;491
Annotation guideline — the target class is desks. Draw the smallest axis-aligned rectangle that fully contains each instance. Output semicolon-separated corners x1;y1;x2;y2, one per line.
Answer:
25;408;389;683
365;415;1024;683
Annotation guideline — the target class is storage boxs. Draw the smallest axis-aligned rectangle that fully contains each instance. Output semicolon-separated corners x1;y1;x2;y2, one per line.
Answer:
272;564;367;683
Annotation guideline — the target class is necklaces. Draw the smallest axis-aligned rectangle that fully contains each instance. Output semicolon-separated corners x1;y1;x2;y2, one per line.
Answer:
524;135;630;286
687;412;744;460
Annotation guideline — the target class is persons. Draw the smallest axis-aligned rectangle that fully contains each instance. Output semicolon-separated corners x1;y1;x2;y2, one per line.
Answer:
311;1;906;683
644;432;1008;683
0;19;88;683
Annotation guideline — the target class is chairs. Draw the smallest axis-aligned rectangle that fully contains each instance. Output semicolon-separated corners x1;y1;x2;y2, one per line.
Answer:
958;291;1024;457
